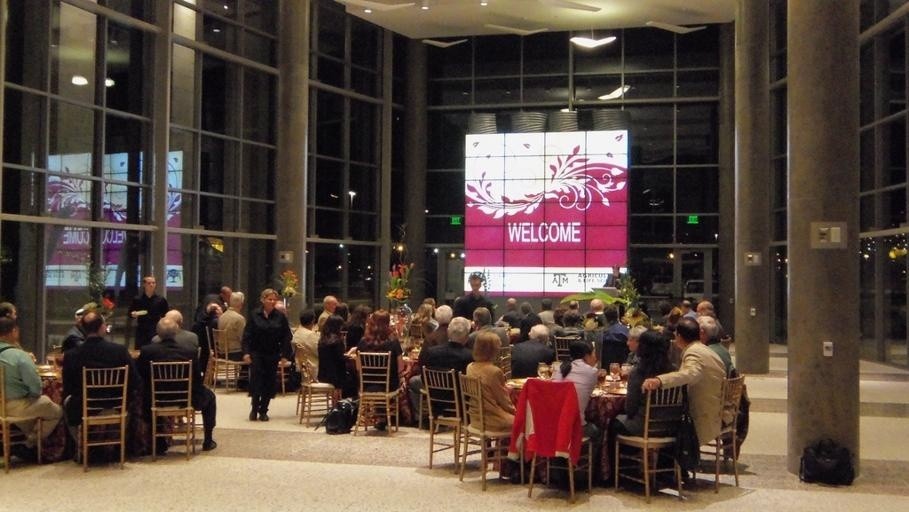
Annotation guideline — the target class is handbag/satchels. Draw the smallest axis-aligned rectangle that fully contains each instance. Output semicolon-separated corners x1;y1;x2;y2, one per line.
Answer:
799;439;854;486
290;371;301;390
326;397;358;434
678;414;701;470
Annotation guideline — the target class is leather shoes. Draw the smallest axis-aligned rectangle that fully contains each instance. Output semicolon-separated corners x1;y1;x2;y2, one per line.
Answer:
250;411;268;421
203;439;217;451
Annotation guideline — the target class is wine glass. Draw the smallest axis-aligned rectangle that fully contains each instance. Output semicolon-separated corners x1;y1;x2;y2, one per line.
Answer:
537;361;632;395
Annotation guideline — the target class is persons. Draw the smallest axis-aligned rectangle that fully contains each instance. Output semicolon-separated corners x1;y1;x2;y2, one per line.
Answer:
198;286;247;361
292;296;733;486
455;274;495;322
128;276;169;350
604;265;625;319
241;289;293;422
1;303;216;465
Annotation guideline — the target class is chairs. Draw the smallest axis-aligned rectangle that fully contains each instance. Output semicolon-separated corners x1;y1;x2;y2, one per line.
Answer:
0;357;197;471
207;324;408;435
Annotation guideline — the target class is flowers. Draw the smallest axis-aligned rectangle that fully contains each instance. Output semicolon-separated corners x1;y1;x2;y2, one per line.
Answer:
382;262;413;301
278;269;300;299
